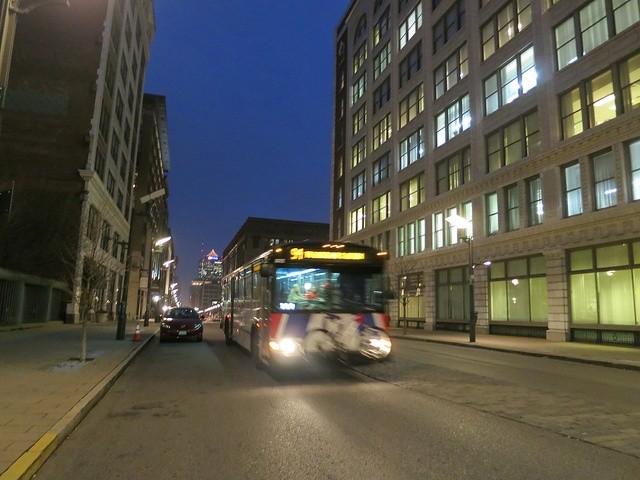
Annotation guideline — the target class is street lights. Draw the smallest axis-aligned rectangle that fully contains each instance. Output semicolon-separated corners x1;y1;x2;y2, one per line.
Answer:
155;259;175;322
144;236;172;327
168;281;178;310
445;214;476;343
116;188;165;341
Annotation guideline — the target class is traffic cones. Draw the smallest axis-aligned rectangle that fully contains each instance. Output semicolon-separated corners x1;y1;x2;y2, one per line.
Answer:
134;324;141;342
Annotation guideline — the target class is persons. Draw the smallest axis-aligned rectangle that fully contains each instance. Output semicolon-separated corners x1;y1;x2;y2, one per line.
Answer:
306;286;320;299
287;284;305;303
321;280;333;298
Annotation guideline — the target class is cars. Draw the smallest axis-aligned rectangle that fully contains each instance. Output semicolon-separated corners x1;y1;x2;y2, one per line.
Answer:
160;307;205;343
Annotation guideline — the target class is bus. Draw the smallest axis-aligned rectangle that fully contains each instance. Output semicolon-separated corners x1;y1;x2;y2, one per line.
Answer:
220;240;392;370
